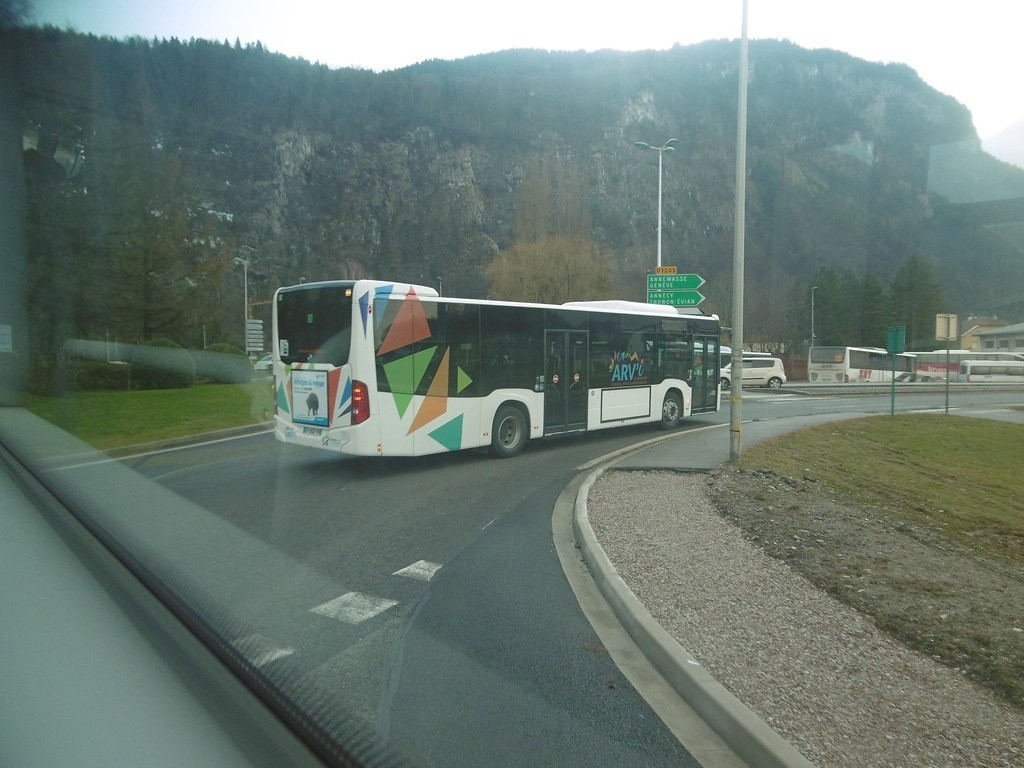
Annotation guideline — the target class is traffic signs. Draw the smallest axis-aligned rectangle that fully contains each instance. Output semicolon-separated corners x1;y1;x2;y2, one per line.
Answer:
646;274;706;305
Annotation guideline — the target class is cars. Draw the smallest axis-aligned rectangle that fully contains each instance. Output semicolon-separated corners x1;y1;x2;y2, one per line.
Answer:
254;356;273;370
721;357;787;388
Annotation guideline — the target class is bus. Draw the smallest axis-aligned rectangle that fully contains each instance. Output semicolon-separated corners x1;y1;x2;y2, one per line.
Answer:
572;340;771;382
808;346;916;383
271;279;720;459
959;359;1024;383
904;348;1024;382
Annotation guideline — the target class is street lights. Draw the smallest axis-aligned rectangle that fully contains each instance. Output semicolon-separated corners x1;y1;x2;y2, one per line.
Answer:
636;138;676;268
235;256;248;355
811;287;818;346
